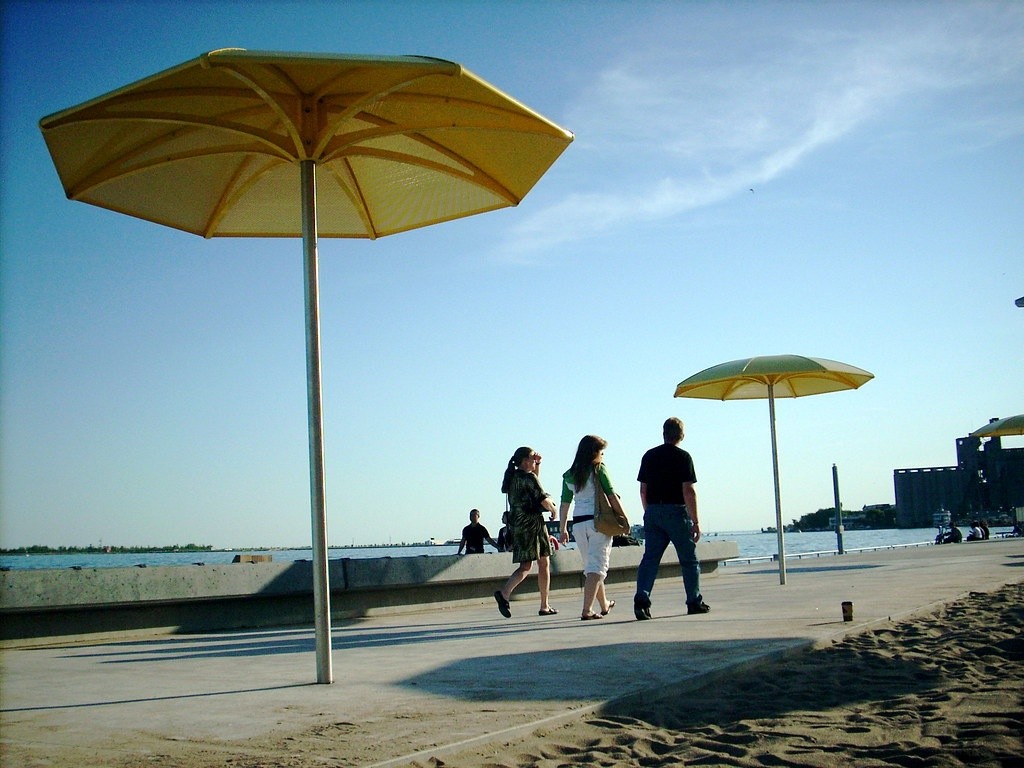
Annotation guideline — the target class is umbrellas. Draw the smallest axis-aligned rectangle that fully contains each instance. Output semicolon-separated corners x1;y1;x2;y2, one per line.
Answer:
674;356;874;584
38;46;576;683
969;414;1024;436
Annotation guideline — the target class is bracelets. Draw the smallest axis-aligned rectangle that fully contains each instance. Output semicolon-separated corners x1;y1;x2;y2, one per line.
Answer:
693;521;698;524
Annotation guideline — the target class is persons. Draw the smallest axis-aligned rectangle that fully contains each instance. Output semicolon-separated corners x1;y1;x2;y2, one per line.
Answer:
1008;524;1024;538
456;508;513;554
559;435;630;621
494;447;557;618
633;417;711;620
943;521;990;544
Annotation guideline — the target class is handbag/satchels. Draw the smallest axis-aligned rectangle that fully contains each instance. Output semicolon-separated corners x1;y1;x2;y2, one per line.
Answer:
502;529;514;547
593;462;630;537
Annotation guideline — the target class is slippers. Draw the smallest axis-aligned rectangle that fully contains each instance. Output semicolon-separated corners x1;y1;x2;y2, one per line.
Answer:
581;613;603;620
494;590;512;618
600;600;616;616
539;607;560;616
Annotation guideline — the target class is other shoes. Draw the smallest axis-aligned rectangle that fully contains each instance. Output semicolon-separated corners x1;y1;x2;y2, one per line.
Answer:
634;603;652;620
687;602;711;614
